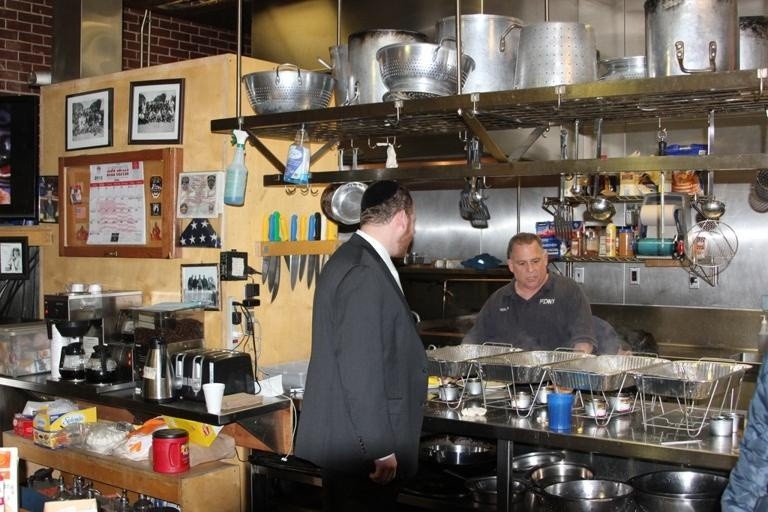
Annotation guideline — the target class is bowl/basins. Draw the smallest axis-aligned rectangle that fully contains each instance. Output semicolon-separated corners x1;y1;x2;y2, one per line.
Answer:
420;434;729;510
332;181;372;226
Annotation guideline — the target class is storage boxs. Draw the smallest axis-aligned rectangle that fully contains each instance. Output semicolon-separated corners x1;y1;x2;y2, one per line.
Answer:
1;320;53;382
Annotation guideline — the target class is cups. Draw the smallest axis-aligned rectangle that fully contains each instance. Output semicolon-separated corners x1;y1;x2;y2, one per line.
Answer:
72;282;103;294
467;378;484;394
203;382;227;413
546;394;573;431
440;384;461;401
508;410;549;430
712;437;741;452
510;387;556;408
711;412;745;437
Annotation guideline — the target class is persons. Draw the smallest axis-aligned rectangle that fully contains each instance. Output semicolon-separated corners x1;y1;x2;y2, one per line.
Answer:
188;275;213;290
718;344;768;512
10;247;21;270
152;221;161;239
461;234;598;356
72;106;101;137
291;180;430;511
140;99;175;124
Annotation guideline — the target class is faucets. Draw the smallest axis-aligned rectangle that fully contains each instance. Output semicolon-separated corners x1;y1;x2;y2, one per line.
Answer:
410;310;422;325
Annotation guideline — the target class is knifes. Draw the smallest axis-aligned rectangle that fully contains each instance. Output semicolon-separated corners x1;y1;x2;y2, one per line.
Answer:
258;209;339;303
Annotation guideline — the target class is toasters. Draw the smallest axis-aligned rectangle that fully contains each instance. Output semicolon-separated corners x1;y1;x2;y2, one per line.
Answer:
169;346;255;400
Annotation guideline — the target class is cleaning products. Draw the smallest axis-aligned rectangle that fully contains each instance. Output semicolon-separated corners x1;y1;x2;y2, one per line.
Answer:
223;129;249;207
280;122;313;184
756;314;768;355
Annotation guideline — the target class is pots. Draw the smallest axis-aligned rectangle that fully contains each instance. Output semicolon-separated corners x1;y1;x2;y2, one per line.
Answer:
328;12;520;104
646;0;768;75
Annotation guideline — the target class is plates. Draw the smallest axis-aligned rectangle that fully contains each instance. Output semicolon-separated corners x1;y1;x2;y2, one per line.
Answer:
457;378;506;389
426;375;447;388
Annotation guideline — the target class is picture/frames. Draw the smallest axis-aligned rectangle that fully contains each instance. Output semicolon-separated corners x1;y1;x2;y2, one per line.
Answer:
0;235;31;281
64;88;113;152
124;78;185;147
178;261;223;312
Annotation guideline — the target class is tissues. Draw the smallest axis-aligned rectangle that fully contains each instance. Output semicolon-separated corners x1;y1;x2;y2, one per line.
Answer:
13;401;50;440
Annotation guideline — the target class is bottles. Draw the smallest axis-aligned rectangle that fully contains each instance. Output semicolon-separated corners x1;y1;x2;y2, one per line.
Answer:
143;339;178;404
608;392;631;411
569;222;634;256
586;398;606;417
154;429;190;473
53;474;153;512
435;258;463;268
584;415;633;439
283;128;310;186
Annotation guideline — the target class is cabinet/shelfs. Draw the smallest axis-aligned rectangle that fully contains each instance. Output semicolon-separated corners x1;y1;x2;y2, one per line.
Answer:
2;425;244;512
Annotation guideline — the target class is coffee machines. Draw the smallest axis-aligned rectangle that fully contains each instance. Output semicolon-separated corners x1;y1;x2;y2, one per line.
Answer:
113;299;207;397
44;291;142;393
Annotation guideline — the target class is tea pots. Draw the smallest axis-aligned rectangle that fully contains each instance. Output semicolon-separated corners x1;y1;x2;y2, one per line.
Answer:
59;342;118;381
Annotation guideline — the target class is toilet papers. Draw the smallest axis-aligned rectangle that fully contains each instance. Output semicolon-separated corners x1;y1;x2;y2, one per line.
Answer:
50;323;81;381
